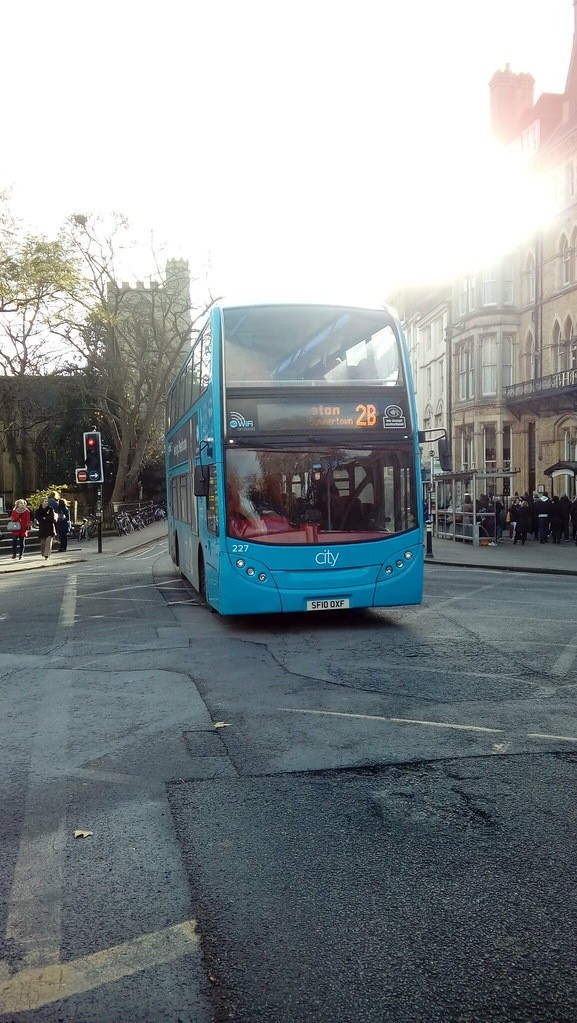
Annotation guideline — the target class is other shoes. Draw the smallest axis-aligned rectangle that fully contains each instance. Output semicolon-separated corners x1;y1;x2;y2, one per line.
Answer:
60;547;65;552
19;556;23;559
12;555;16;558
513;539;517;544
535;536;577;544
522;540;525;545
488;542;497;546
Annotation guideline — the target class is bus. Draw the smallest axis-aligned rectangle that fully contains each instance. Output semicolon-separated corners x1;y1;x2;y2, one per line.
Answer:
164;300;453;615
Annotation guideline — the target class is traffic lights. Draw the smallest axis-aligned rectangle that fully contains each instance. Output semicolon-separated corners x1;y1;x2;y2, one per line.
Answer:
102;449;114;482
75;432;103;484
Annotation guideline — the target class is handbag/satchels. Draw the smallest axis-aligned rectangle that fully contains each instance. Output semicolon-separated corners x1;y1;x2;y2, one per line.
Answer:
7;517;21;531
47;508;59;523
506;511;510;522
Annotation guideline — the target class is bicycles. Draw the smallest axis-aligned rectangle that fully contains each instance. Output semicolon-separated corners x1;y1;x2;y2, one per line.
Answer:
112;500;167;536
78;514;96;542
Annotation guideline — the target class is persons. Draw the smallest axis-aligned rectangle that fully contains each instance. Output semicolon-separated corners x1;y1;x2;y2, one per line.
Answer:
36;491;70;560
306;468;341;524
352;358;375;380
442;495;453;532
425;497;435;517
462;492;577;546
11;499;31;559
